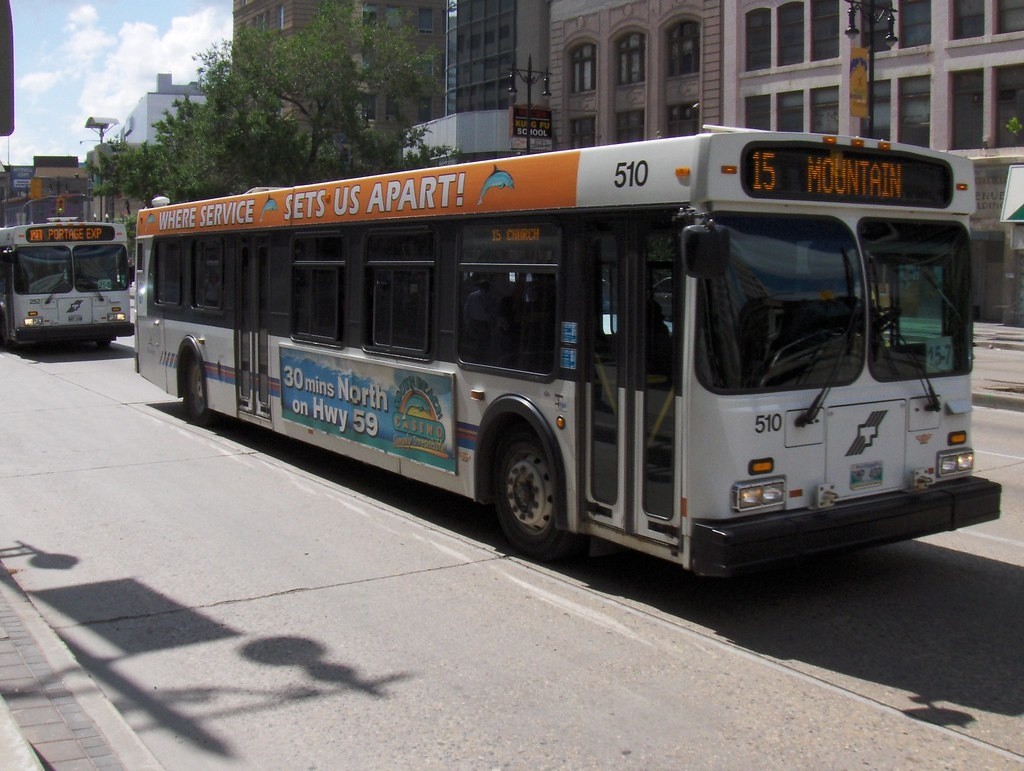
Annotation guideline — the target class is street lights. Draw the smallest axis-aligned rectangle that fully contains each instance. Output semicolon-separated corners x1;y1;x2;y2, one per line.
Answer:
843;0;900;139
503;52;555;155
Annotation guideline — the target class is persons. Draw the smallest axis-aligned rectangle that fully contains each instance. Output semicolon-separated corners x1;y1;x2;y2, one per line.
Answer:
206;263;583;356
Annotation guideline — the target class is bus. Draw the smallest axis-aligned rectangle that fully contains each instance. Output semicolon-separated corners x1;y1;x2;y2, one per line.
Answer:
129;121;1005;579
0;216;135;349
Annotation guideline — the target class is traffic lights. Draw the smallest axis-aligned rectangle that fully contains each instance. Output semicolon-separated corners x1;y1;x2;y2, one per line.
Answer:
56;198;65;215
